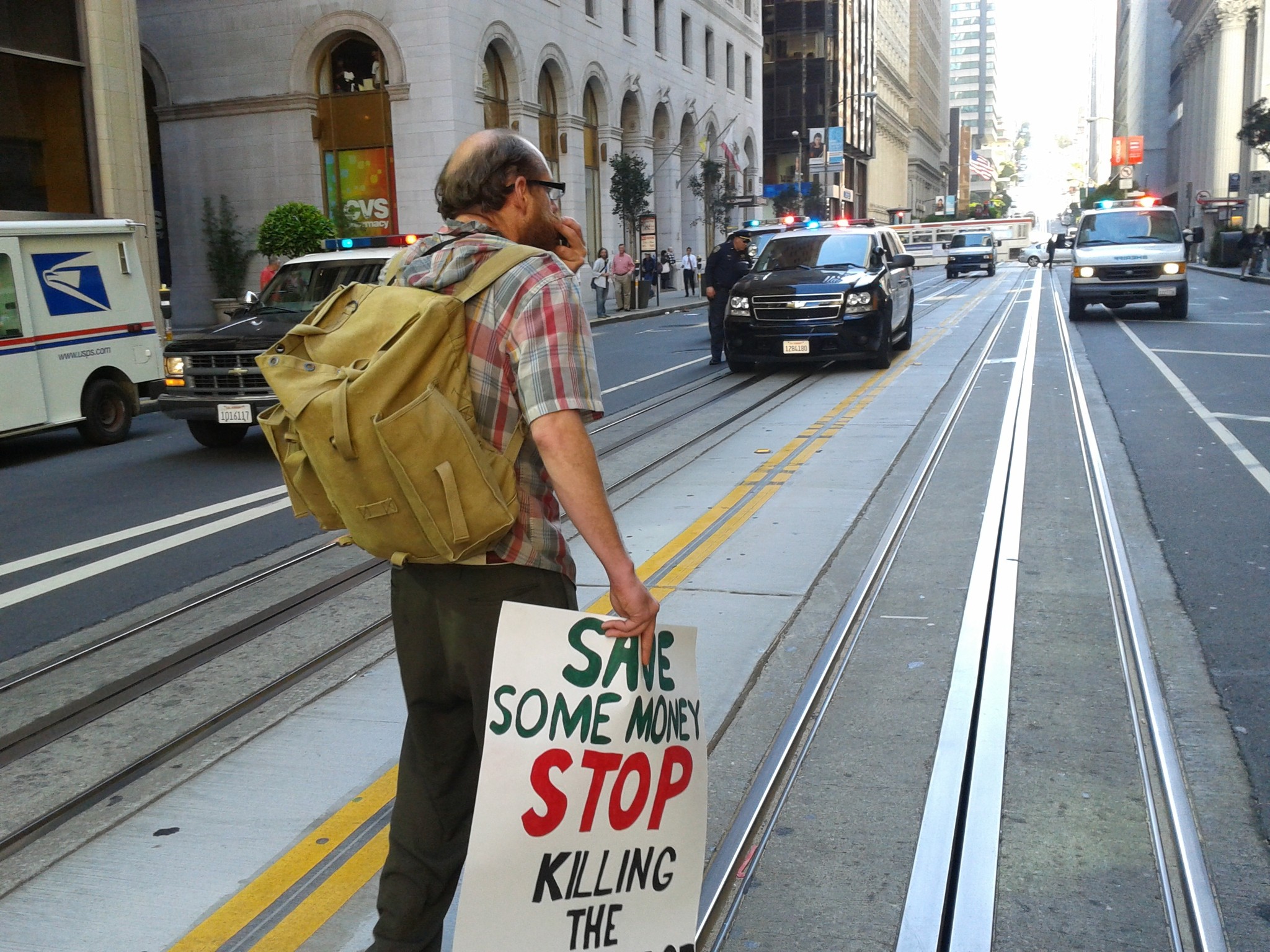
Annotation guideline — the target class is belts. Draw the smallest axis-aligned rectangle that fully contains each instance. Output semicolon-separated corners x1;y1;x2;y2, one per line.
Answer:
616;273;627;276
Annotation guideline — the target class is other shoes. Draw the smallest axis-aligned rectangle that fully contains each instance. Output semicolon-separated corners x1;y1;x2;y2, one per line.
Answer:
605;314;610;317
616;308;623;311
685;295;689;297
625;308;630;312
598;315;606;319
710;357;721;365
692;290;695;295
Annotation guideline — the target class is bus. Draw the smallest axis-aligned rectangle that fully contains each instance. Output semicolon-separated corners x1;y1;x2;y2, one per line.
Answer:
886;218;1032;268
1;221;165;446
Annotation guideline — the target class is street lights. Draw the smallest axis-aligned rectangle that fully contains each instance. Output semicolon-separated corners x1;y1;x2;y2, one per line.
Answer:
1067;179;1087;197
944;163;970;221
1086;118;1129;200
792;131;804;216
824;92;876;220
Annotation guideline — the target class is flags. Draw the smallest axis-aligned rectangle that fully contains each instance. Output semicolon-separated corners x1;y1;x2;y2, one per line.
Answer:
723;120;750;174
699;109;712;159
970;151;993;181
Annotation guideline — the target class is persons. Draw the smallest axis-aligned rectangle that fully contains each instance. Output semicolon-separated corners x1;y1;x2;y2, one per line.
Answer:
642;252;655;284
1239;224;1270;282
681;247;697;297
371;49;389;89
280;270;307;294
333;57;352;91
1043;233;1055;269
260;256;282;306
936;199;943;211
592;247;612;318
611;244;636;311
1183;225;1193;261
660;246;676;292
705;229;754;364
810;133;825;158
367;127;660;952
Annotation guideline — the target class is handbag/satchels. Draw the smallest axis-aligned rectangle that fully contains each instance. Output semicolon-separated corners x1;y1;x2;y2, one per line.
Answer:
590;277;596;288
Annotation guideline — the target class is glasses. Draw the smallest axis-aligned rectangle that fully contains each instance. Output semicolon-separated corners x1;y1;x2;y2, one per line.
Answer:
501;179;566;200
737;237;749;243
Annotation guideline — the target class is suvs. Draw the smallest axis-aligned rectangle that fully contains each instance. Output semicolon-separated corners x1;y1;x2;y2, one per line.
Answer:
723;219;914;373
1055;198;1204;320
149;233;432;449
941;228;1000;279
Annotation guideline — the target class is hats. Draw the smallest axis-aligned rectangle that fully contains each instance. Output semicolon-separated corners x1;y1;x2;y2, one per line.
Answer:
734;229;752;242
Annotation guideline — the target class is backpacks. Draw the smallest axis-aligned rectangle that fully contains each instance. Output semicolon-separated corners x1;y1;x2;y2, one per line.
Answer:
254;243;538;567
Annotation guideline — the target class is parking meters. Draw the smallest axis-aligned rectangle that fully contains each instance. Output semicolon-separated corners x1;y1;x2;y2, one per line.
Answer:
696;255;702;299
634;260;641;311
158;284;173;347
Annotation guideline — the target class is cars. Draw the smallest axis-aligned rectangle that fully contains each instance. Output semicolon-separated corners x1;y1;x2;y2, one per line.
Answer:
1015;242;1071;267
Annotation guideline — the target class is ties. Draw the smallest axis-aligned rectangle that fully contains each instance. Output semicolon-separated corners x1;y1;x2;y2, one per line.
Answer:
687;256;692;269
